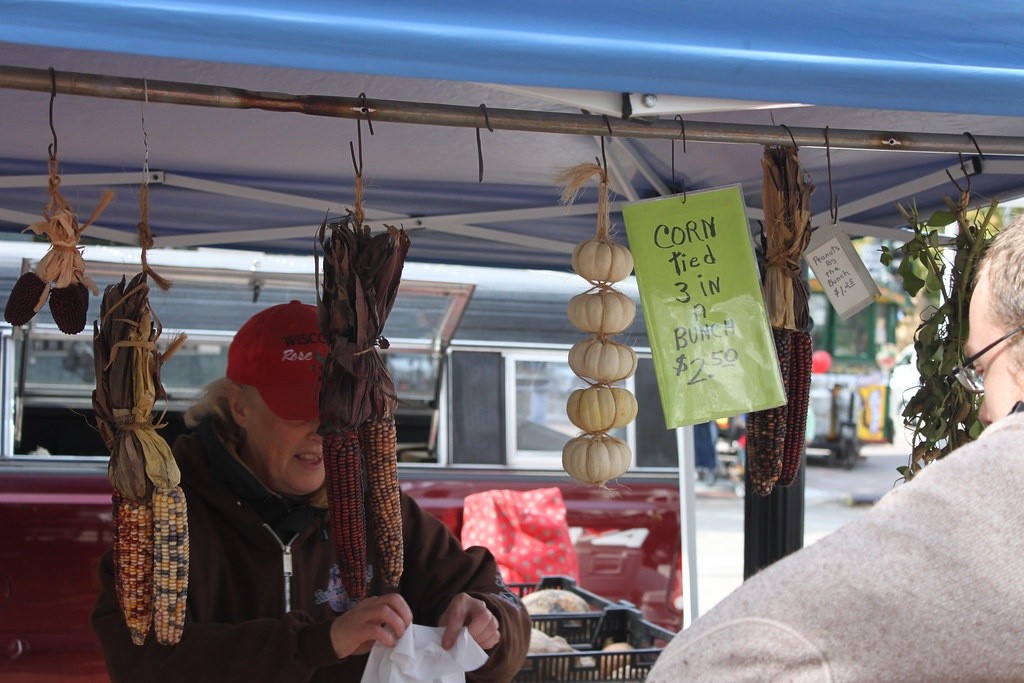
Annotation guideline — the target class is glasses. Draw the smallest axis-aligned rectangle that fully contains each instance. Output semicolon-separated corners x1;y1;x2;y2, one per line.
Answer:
952;325;1024;393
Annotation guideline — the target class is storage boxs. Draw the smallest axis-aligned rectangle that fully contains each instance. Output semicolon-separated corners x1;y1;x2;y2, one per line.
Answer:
504;574;678;683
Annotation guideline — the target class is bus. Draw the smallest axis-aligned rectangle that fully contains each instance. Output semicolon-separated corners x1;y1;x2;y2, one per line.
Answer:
803;274;907;468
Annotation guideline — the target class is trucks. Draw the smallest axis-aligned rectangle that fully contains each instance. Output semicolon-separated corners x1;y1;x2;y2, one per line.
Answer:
0;235;682;681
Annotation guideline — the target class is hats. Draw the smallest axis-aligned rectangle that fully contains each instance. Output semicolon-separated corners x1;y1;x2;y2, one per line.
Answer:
225;300;330;420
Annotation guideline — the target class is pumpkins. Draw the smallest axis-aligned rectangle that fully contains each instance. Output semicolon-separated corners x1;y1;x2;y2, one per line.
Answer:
568;292;636;334
570;239;635;283
568;340;638;382
522;587;651;680
566;386;639;432
562;436;631;483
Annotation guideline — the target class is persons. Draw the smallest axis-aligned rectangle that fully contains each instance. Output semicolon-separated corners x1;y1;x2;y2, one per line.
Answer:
640;213;1024;683
88;298;532;683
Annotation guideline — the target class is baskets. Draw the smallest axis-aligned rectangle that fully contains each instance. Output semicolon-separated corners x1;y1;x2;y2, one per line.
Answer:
505;575;678;683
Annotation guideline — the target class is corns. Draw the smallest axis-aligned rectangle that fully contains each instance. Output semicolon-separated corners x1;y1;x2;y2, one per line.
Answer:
321;430;369;601
4;272;50;327
49;282;89;334
112;485;190;646
746;326;814;497
357;417;403;589
